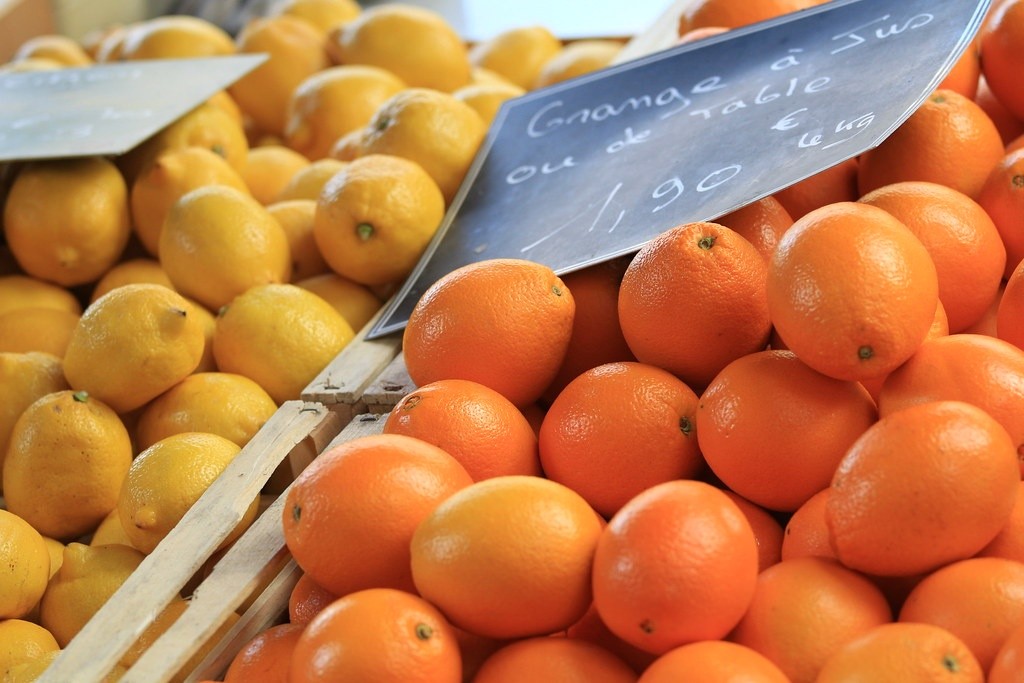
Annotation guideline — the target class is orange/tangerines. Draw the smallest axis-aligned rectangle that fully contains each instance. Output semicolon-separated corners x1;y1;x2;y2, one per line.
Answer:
227;0;1024;683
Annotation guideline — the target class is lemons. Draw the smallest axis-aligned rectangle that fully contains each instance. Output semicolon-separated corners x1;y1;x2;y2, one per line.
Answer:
0;0;626;683
